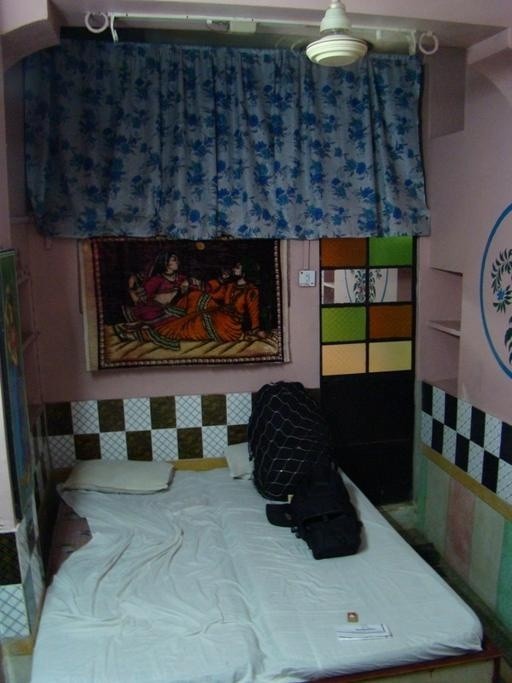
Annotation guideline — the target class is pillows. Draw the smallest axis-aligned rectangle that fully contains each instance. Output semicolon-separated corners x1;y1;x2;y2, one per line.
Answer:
60;458;176;493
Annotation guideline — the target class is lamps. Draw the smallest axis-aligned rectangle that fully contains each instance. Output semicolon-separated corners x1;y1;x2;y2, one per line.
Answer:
303;0;370;68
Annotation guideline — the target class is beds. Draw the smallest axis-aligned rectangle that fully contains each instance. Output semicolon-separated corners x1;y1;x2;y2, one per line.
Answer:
33;451;501;682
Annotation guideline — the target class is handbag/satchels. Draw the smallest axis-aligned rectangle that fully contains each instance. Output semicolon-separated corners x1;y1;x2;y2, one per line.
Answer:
297;500;362;560
246;381;338;500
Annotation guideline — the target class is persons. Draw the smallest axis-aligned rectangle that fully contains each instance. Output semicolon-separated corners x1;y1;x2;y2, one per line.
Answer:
113;247;273;350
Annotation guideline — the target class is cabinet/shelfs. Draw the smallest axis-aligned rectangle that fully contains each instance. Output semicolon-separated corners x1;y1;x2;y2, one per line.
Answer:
412;25;511;639
0;246;54;658
